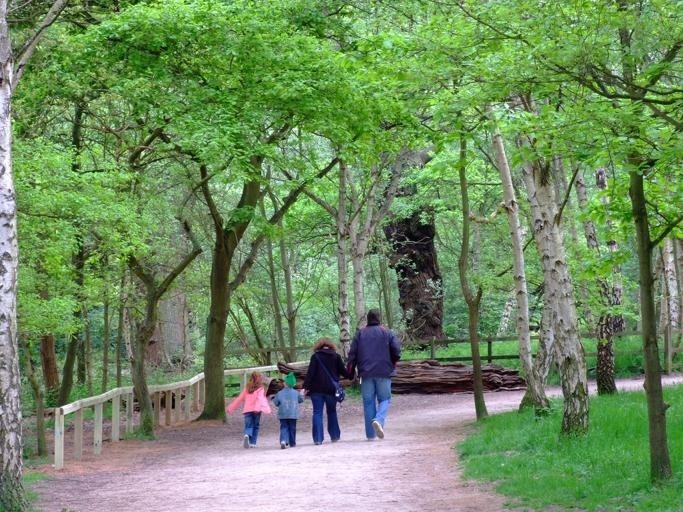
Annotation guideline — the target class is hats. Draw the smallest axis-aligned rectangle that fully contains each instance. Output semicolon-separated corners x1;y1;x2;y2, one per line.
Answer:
284;371;297;388
367;309;380;322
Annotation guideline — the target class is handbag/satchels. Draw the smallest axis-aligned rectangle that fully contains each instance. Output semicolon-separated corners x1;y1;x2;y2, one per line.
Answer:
335;381;344;403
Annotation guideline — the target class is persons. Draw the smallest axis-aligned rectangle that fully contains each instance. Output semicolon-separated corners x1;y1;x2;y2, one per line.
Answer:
301;337;355;445
272;372;306;449
225;371;270;448
345;309;401;441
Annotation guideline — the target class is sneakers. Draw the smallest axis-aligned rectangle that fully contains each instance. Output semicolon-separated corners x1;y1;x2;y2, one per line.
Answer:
332;439;337;442
243;435;256;448
372;421;384;438
280;441;295;449
314;441;322;445
368;437;376;441
